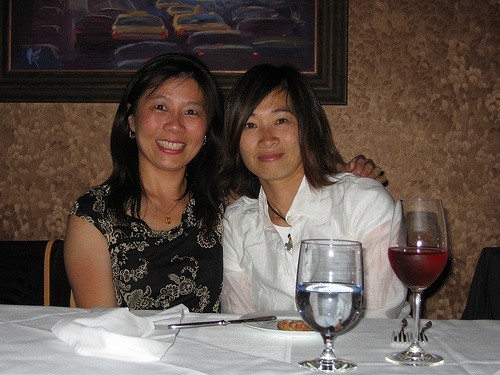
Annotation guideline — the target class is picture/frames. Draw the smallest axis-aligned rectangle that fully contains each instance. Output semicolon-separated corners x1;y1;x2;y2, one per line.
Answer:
0;0;349;106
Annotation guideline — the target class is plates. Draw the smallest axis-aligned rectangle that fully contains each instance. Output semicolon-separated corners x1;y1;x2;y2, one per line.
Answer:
240;310;322;342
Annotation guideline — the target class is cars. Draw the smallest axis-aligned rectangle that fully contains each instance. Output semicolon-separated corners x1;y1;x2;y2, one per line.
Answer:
13;1;308;69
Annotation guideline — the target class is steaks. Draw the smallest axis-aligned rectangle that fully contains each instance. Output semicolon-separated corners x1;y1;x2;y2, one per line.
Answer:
277;320;313;331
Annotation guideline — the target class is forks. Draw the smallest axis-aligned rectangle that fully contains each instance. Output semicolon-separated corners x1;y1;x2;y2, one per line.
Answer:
418;321;432;342
391;318;410;344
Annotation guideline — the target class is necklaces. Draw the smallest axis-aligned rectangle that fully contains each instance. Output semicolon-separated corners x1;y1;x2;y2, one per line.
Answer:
266;199;294;252
142;193;185;224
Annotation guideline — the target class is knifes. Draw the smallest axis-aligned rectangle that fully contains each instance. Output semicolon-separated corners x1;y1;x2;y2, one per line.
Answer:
168;316;277;329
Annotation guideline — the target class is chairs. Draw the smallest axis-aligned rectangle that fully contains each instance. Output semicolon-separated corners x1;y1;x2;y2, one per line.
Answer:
1;239;72;308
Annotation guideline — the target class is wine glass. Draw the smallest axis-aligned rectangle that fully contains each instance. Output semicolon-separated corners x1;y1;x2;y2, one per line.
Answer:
295;239;364;374
385;198;448;367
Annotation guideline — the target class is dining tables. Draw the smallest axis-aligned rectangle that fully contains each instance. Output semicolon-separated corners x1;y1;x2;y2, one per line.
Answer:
0;303;500;374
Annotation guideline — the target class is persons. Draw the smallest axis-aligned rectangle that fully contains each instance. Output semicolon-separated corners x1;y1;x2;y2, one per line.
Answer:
63;53;389;313
217;61;413;320
